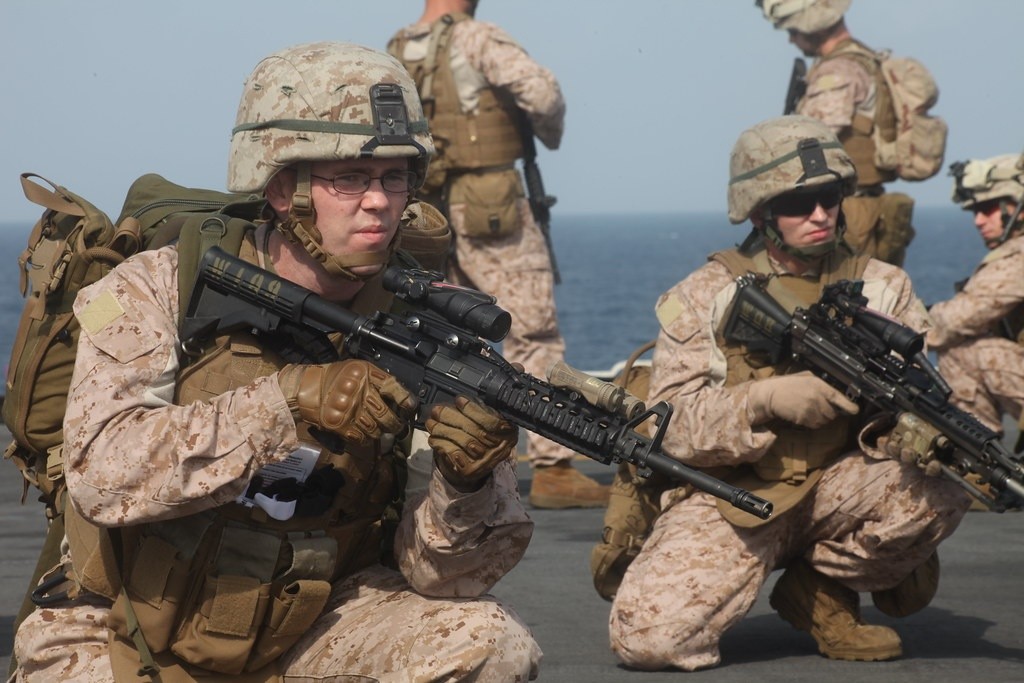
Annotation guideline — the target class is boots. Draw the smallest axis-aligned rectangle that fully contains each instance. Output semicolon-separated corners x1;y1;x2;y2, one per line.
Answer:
530;459;610;508
769;558;904;662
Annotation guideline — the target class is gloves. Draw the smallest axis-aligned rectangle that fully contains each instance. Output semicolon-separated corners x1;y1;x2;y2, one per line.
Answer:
875;436;943;480
745;369;860;430
425;362;525;491
278;358;418;447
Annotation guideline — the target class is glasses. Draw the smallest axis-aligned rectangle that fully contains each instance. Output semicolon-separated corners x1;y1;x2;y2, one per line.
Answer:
972;197;1008;216
286;165;423;195
763;182;845;216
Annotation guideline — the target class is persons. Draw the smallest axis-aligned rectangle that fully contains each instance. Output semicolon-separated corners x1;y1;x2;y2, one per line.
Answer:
925;154;1024;512
757;1;891;260
373;0;613;510
611;116;974;670
0;41;544;683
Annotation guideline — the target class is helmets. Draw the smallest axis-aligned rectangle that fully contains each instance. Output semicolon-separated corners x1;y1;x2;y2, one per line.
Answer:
757;0;850;33
228;41;435;193
948;154;1024;214
727;115;859;223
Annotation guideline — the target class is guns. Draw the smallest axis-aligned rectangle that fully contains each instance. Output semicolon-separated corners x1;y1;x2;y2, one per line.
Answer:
179;244;777;523
721;269;1024;515
514;109;561;286
784;58;807;115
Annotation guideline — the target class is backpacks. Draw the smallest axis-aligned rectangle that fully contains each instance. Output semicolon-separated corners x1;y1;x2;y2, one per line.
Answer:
827;44;948;183
0;172;453;524
